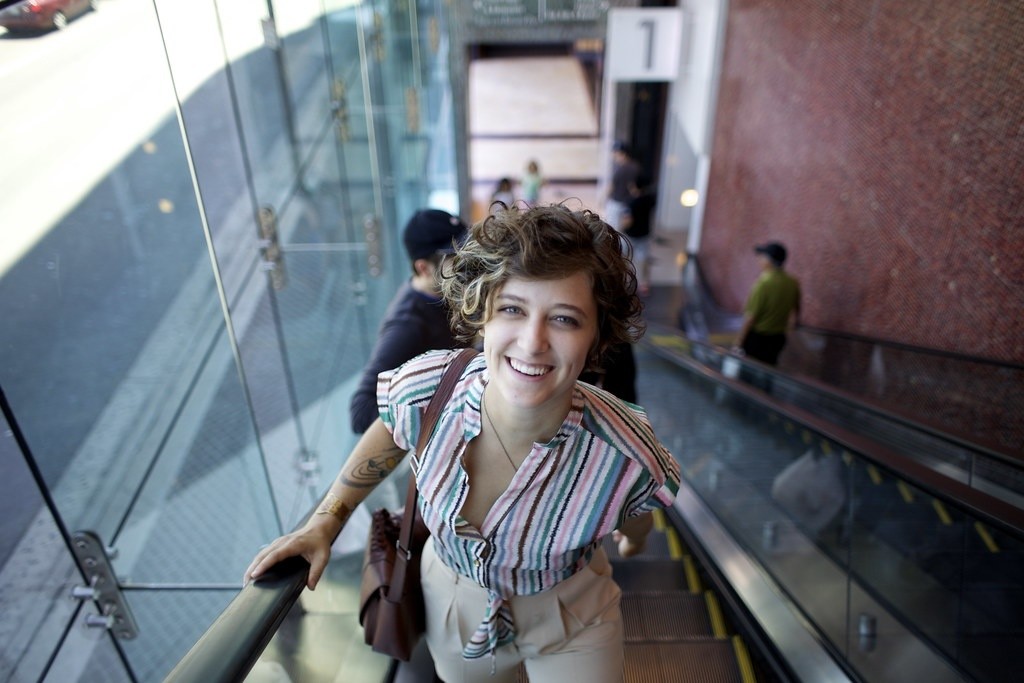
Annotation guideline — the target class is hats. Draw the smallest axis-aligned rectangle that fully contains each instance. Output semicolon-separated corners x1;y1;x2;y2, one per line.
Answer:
753;243;787;265
403;210;469;258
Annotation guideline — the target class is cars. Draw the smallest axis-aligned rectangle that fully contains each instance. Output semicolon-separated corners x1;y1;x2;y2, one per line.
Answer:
0;0;98;30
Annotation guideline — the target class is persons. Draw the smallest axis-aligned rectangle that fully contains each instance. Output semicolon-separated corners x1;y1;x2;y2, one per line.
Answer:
489;178;515;219
243;197;684;683
520;162;545;208
594;140;651;235
735;240;804;394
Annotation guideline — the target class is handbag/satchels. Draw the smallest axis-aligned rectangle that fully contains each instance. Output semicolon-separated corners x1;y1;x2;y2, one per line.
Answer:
358;504;431;661
